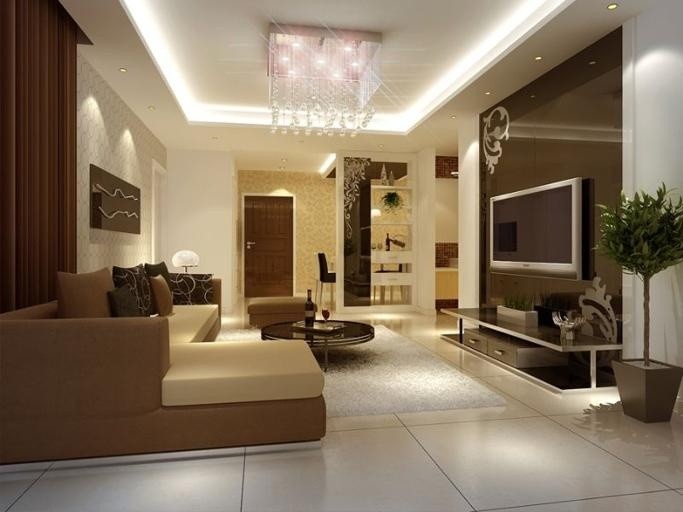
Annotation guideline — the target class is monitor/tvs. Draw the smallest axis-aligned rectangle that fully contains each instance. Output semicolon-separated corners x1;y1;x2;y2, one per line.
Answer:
489;176;582;282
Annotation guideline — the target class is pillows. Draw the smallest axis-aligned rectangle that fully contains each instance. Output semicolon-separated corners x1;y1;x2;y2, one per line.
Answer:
53;261;213;319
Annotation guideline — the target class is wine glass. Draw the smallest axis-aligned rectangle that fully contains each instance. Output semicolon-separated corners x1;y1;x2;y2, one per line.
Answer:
371;240;382;251
320;305;330;326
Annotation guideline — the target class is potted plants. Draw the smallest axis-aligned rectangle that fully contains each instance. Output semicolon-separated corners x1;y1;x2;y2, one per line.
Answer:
497;293;539;321
591;180;683;421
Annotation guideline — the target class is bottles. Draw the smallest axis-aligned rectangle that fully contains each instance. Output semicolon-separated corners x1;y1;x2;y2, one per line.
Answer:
380;163;394;185
385;232;405;251
303;289;314;327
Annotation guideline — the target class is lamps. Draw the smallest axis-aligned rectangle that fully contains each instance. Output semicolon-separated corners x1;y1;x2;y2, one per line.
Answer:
172;249;200;273
265;23;383;138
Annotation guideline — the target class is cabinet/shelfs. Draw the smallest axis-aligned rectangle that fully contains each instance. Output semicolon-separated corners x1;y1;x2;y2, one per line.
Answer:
440;308;623;396
370;185;414;302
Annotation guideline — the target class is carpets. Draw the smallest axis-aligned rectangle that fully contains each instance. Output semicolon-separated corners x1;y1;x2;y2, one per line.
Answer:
212;323;508;419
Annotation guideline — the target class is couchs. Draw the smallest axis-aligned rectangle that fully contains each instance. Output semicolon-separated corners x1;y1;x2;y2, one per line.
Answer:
0;279;326;465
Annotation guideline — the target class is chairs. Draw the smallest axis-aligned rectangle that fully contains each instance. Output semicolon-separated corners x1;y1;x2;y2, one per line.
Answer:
374;264;405;304
315;252;335;306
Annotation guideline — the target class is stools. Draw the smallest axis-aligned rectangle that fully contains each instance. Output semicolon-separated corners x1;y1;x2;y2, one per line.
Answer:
245;295;316;328
